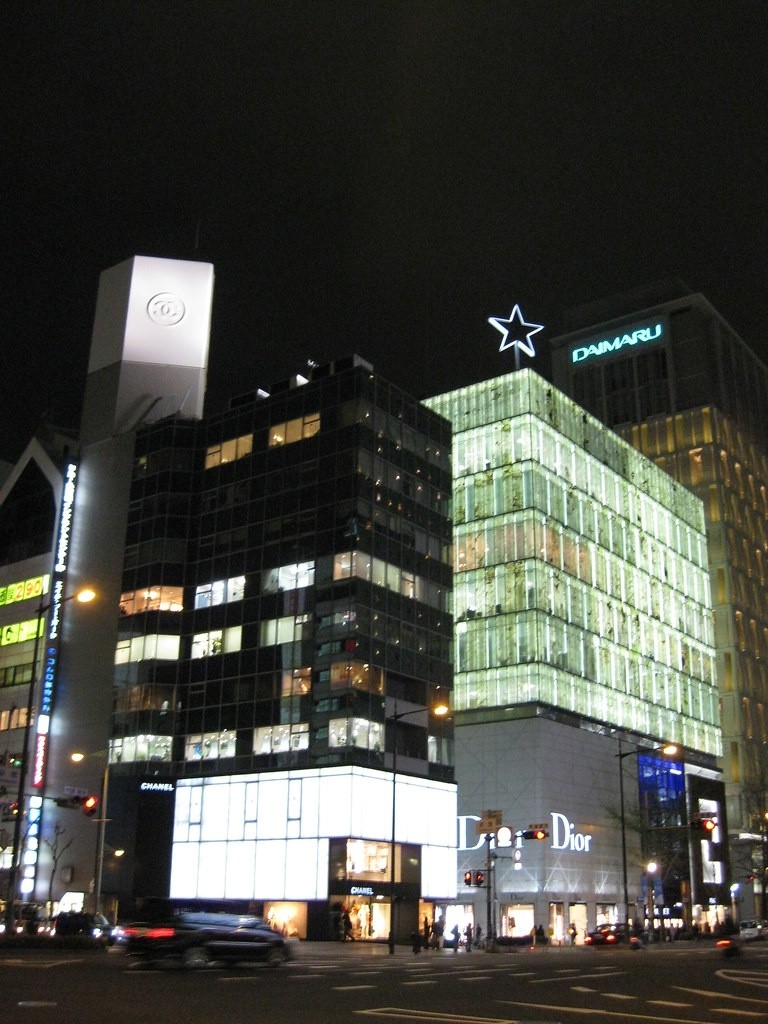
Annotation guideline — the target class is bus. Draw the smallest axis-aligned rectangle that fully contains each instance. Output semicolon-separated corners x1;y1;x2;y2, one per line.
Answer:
11;899;47;937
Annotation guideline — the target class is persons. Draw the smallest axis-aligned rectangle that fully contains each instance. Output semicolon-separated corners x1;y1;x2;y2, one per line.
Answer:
340;907;736;951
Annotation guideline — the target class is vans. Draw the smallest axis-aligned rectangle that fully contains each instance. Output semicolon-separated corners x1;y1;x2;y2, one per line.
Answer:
740;918;768;941
55;911;117;949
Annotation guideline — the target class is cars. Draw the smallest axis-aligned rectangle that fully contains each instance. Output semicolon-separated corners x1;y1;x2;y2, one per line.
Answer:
584;923;642;946
122;910;296;971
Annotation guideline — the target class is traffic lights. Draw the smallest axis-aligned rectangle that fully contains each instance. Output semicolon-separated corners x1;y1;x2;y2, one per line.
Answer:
695;820;715;831
523;830;546;840
83;796;98;818
476;872;484;887
464;872;472;886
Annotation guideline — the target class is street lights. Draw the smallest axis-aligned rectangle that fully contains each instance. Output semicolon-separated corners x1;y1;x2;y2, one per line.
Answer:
388;704;449;953
71;745;111;913
619;744;678;932
